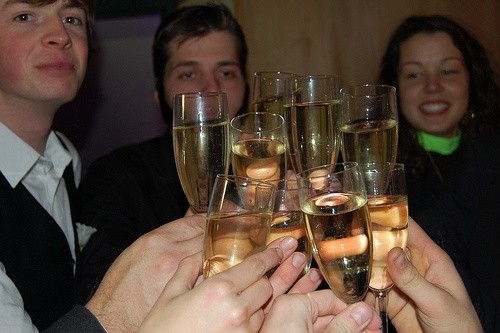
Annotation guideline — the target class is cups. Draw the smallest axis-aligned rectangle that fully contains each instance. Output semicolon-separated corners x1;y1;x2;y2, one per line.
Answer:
202;174;275;280
284;75;341;174
172;93;229;213
253;71;294;116
297;161;373;333
339;84;398;195
258;180;312;295
228;111;287;214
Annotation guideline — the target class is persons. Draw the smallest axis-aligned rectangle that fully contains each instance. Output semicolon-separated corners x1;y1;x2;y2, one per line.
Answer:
307;15;500;333
0;215;484;333
0;0;95;333
79;2;283;309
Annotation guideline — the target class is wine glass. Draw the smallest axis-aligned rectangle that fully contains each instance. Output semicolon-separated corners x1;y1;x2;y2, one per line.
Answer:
343;162;409;333
